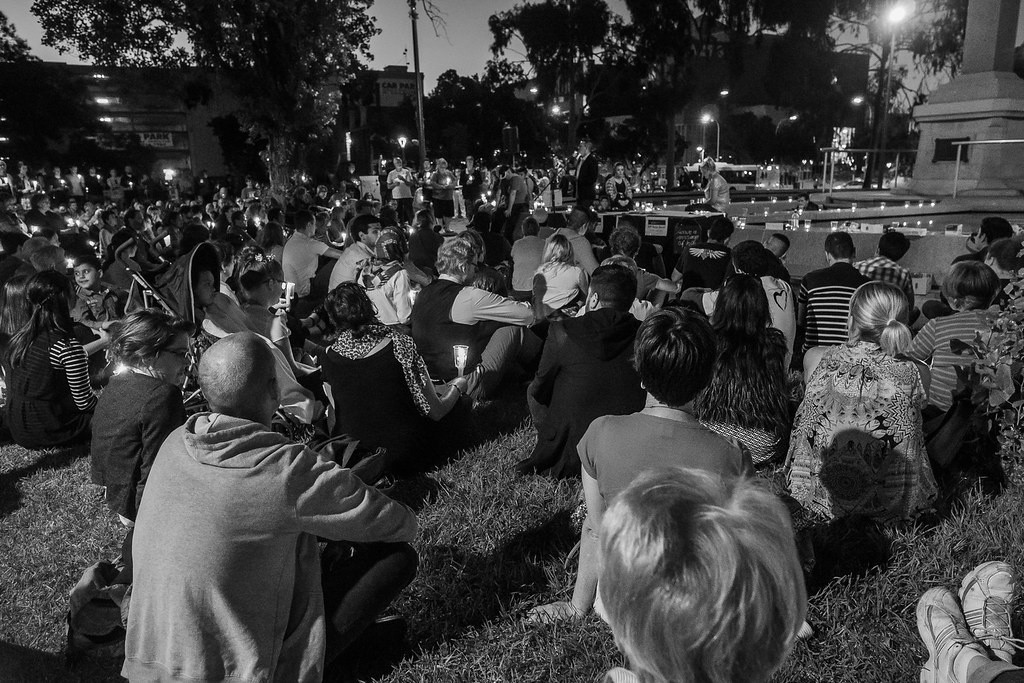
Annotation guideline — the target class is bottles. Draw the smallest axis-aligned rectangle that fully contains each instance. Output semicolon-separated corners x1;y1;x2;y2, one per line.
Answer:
690;198;705;205
791;209;799;231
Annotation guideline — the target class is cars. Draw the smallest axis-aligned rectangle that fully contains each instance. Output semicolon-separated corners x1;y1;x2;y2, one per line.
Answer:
834;178;873;190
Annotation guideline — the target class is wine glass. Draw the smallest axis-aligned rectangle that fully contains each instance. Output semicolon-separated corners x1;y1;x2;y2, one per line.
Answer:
453;345;469;378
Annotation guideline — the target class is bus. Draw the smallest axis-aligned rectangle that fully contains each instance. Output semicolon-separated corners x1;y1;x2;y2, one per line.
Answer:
683;162;781;191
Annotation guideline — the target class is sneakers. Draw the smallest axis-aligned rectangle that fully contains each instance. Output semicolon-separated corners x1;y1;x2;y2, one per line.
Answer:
957;560;1024;665
916;587;990;683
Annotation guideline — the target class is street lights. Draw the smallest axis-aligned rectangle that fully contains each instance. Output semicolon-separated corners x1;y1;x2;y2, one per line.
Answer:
853;96;873;178
702;114;721;162
773;115;798;164
875;4;910;191
396;135;408;167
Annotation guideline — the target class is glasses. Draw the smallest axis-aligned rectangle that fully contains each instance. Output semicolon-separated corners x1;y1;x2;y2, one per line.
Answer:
262;279;288;290
160;348;190;360
471;263;480;273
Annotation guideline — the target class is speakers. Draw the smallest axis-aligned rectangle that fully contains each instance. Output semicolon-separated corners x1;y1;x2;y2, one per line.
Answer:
502;126;519;155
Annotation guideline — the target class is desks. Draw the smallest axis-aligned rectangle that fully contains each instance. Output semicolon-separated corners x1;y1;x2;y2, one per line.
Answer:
492;208;726;264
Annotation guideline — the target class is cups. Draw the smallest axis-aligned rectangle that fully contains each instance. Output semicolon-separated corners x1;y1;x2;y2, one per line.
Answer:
798;206;804;215
663;200;667;209
851;203;857;212
773;197;777;204
739;218;746;229
804;220;811;232
930;199;936;208
904;200;911;208
788;196;793;203
286;282;295;299
892;221;899;228
818;205;823;212
731;216;738;228
831;221;838;232
640;202;645;209
751;197;755;204
881;202;886;211
918;199;924;208
763;206;769;218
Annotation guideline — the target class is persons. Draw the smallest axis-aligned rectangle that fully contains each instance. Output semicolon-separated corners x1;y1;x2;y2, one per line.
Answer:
685;155;731;212
118;332;417;683
336;151;560;233
795;193;819;210
913;561;1023;683
1;157;243;527
593;465;809;683
570;138;600;213
505;218;815;639
164;183;541;470
789;210;1024;528
604;161;636;210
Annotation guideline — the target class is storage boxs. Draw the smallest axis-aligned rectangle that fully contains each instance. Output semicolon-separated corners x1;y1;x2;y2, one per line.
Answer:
911;273;932;295
